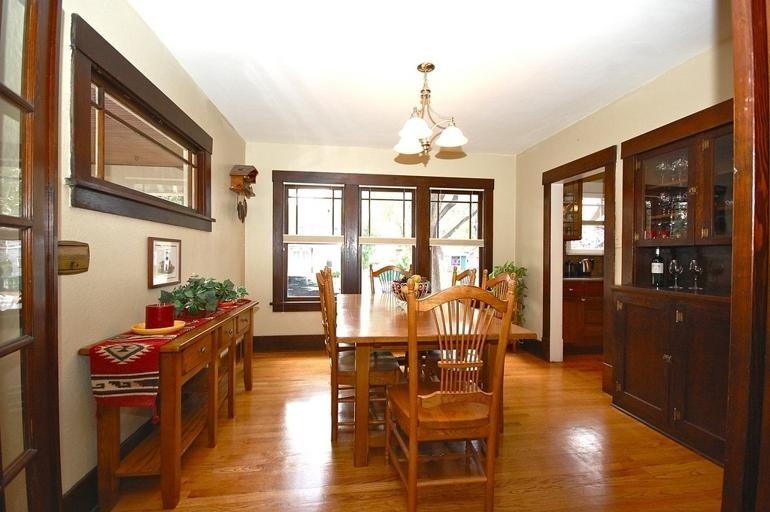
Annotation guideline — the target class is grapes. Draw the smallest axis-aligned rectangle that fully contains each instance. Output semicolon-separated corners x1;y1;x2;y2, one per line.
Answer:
391;276;406;302
418;277;427;297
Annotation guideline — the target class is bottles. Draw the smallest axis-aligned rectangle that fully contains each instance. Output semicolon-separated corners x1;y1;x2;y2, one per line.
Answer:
649;246;666;288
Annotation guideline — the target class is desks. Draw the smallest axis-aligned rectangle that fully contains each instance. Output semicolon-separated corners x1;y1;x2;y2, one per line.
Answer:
335;293;539;469
77;297;260;512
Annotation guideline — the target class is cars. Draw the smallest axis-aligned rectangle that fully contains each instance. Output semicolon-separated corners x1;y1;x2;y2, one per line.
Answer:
287;275;320;296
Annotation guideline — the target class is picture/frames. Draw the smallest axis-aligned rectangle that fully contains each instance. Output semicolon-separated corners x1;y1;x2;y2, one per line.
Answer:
146;237;182;290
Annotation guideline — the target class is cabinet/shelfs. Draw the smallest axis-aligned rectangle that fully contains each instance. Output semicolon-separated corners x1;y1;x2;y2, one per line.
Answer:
609;97;735;472
562;180;583;240
563;281;604;355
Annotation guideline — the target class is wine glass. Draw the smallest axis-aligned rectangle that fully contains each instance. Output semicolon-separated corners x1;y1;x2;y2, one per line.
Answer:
654;156;689;185
687;259;704;291
667;259;685;290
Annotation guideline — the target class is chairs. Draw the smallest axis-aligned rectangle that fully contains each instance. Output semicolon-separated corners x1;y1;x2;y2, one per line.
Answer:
367;262;418;292
384;273;517;512
423;265;517;435
313;263;401;469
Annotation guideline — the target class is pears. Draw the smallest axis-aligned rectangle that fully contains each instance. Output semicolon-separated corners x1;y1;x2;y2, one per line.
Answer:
402;274;420;293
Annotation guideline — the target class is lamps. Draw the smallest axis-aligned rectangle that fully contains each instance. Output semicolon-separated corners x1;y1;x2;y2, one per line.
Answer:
390;62;469;158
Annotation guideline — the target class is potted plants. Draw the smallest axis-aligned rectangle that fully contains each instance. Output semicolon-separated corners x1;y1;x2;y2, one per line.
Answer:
160;274;250;321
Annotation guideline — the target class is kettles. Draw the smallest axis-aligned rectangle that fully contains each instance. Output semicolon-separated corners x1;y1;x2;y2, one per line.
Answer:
576;257;595;276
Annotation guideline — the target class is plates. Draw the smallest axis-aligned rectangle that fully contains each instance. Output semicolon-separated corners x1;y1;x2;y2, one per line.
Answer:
131;320;186;335
